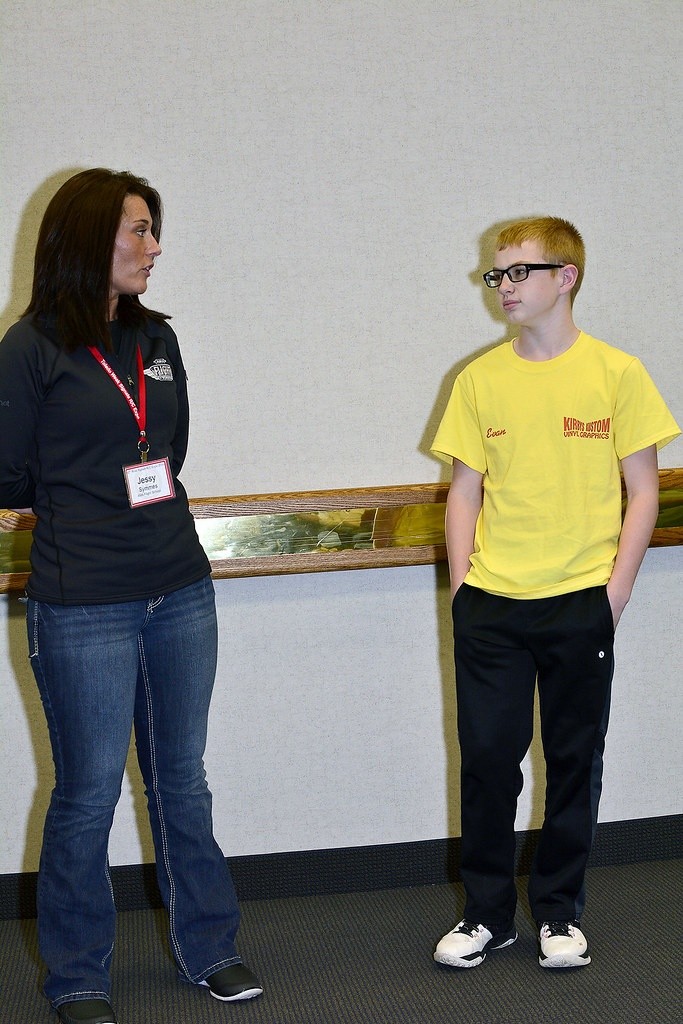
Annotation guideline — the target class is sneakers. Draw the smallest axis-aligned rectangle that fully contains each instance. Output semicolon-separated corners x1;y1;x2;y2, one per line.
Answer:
178;963;263;1001
434;919;518;968
58;999;119;1024
537;920;591;967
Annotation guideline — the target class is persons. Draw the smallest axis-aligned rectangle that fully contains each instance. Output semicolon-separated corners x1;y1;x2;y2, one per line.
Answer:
434;216;659;967
0;167;263;1024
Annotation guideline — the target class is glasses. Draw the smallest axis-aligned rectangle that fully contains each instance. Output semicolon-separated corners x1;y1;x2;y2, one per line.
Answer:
483;264;565;288
